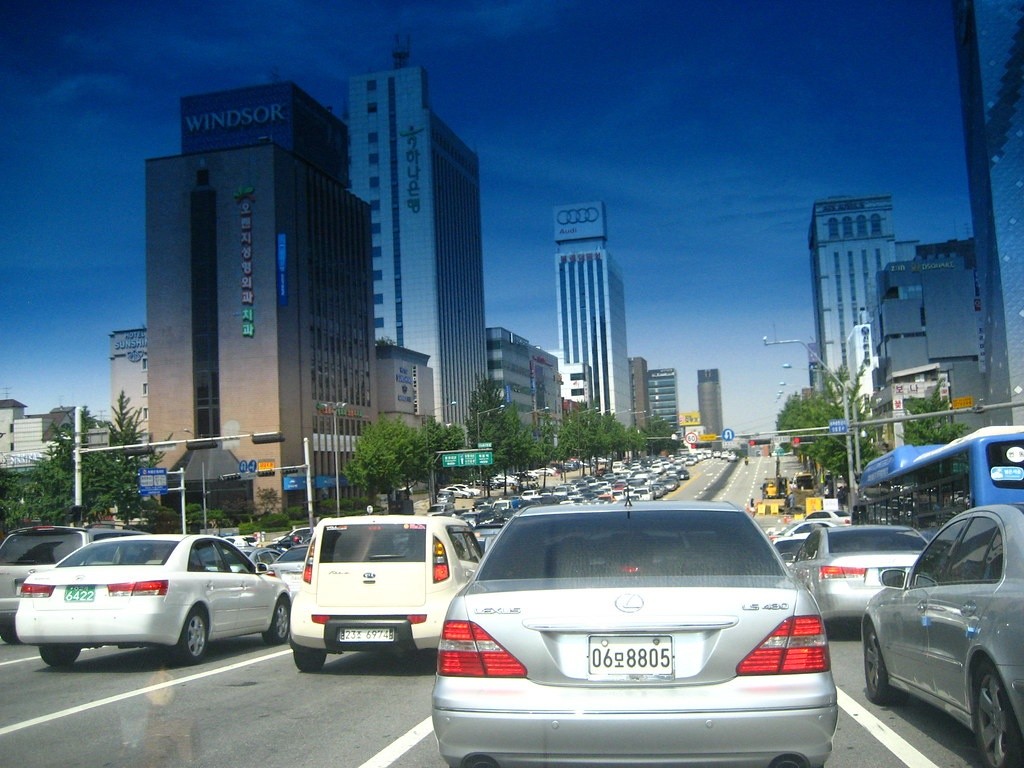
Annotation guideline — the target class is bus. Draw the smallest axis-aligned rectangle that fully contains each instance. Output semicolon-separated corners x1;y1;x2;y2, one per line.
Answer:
851;426;1024;555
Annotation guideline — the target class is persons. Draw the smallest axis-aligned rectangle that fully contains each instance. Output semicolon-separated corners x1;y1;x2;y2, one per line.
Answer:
786;481;797;508
824;475;834;498
766;483;778;499
750;498;755;517
837;486;846;510
511;480;523;494
191;519;200;534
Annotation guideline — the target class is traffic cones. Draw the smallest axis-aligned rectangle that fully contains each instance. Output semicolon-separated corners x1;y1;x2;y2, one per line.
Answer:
523;405;550;492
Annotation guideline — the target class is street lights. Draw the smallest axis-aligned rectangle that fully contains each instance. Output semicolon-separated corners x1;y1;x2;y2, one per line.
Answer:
320;400;349;517
477;404;506;498
576;407;599;479
425;400;457;506
760;335;857;509
183;427;207;535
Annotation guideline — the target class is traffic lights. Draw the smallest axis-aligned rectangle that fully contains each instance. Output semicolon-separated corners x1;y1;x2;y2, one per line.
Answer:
795;437;816;444
281;469;298;475
689;443;713;448
257;471;276;478
223;474;242;481
749;439;771;446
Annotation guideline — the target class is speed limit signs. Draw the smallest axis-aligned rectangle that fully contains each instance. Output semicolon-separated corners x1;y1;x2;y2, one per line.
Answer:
685;432;698;444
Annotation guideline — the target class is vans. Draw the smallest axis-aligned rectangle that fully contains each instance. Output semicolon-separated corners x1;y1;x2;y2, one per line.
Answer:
288;513;484;673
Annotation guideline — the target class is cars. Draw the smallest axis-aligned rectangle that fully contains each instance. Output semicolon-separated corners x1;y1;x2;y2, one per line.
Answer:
14;532;292;670
0;520;159;645
430;499;838;768
857;501;1024;768
765;508;853;573
223;525;315;585
424;446;739;557
780;522;934;638
